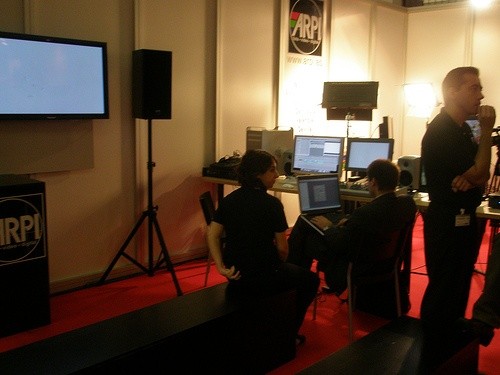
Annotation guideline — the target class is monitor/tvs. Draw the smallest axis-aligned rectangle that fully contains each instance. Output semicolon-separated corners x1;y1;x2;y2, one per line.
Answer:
346;137;394;173
291;134;345;178
0;31;109;119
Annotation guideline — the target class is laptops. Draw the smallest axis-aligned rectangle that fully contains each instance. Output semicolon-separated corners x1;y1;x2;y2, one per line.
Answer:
297;174;346;235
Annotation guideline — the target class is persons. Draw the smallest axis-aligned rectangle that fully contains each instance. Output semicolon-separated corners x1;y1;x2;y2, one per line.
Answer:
208;149;320;345
418;67;496;375
286;159;415;302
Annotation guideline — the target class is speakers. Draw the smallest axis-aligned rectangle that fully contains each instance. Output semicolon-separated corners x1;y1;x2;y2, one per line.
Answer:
132;49;173;119
397;155;422;190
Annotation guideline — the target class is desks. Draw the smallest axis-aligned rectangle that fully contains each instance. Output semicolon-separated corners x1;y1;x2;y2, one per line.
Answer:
199;174;500;321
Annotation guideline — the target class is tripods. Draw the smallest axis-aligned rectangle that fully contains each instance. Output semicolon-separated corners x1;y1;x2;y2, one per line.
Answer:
99;118;183;296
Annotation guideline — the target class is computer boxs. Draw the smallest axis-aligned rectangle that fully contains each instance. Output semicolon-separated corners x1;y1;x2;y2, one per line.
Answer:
246;126;294;176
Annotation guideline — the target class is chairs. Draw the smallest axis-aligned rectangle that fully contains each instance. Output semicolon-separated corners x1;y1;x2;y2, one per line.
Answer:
312;209;422;346
